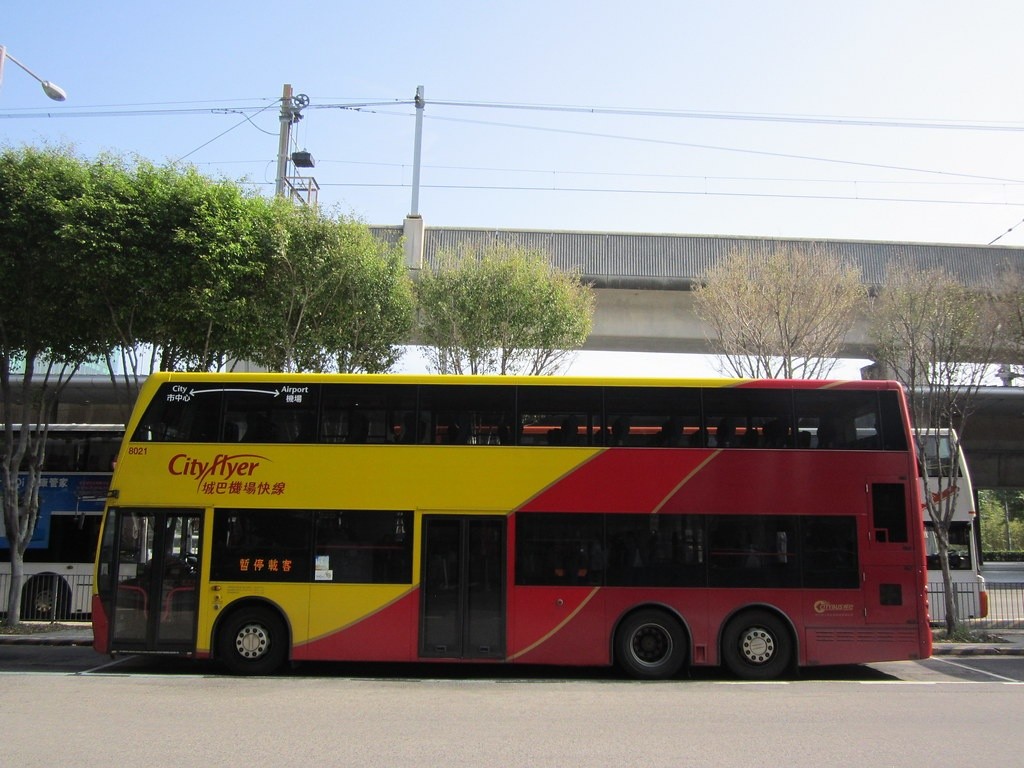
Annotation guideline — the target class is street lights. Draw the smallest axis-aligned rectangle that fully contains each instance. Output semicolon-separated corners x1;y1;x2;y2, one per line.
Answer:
0;44;67;102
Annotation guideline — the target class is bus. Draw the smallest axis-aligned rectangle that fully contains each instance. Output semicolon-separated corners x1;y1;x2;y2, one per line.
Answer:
390;424;988;621
74;372;933;680
0;423;199;621
